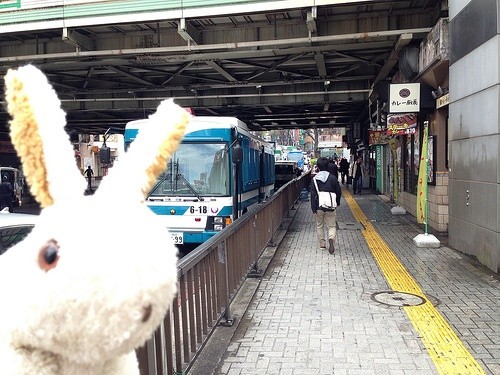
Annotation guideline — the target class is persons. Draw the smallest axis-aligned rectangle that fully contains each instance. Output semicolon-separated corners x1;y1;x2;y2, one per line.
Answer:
85;166;93;184
348;156;365;195
327;156;350;185
310;157;342;254
0;176;13;212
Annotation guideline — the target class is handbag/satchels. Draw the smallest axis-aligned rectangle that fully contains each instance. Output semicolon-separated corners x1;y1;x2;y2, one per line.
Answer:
319;191;337;210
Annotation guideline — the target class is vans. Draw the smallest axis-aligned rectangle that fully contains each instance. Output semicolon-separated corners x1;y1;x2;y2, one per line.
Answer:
0;165;24;210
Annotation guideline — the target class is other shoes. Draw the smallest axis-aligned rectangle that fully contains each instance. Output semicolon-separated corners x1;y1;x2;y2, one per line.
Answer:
320;243;326;249
328;239;335;254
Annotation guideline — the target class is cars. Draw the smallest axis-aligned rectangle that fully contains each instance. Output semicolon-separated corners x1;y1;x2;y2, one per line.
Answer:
268;144;311;193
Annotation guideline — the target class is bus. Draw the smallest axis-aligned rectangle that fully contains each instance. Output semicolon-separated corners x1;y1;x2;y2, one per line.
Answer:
122;114;275;245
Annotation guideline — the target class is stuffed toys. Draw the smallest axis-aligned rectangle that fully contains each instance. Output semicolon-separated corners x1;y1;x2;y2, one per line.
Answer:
0;65;192;375
